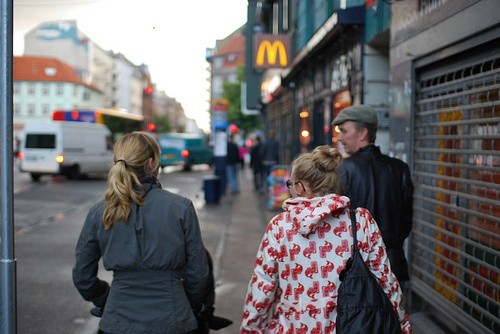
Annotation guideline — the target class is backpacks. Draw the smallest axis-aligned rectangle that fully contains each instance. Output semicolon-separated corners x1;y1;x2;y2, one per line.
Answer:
336;211;402;334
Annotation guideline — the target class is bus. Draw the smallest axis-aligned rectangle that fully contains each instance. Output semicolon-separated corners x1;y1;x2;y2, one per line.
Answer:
52;107;146;138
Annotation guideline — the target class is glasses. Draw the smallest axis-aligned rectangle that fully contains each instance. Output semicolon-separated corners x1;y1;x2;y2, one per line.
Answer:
285;180;306;192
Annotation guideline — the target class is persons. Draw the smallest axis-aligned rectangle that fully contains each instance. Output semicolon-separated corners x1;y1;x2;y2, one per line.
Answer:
226;133;240;193
239;146;412;334
249;136;266;190
261;130;279;176
238;145;249;169
71;132;211;334
331;104;414;291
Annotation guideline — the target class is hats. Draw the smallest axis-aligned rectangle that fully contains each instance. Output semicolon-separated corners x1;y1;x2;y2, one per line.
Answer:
331;105;378;125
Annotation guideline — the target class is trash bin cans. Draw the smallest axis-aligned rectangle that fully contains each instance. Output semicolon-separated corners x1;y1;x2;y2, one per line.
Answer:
203;175;221;204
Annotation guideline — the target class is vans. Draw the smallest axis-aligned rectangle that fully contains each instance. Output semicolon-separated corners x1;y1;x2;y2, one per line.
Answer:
158;131;211;171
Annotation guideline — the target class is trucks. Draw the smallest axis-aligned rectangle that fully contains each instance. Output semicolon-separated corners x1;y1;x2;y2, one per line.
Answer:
17;120;117;181
